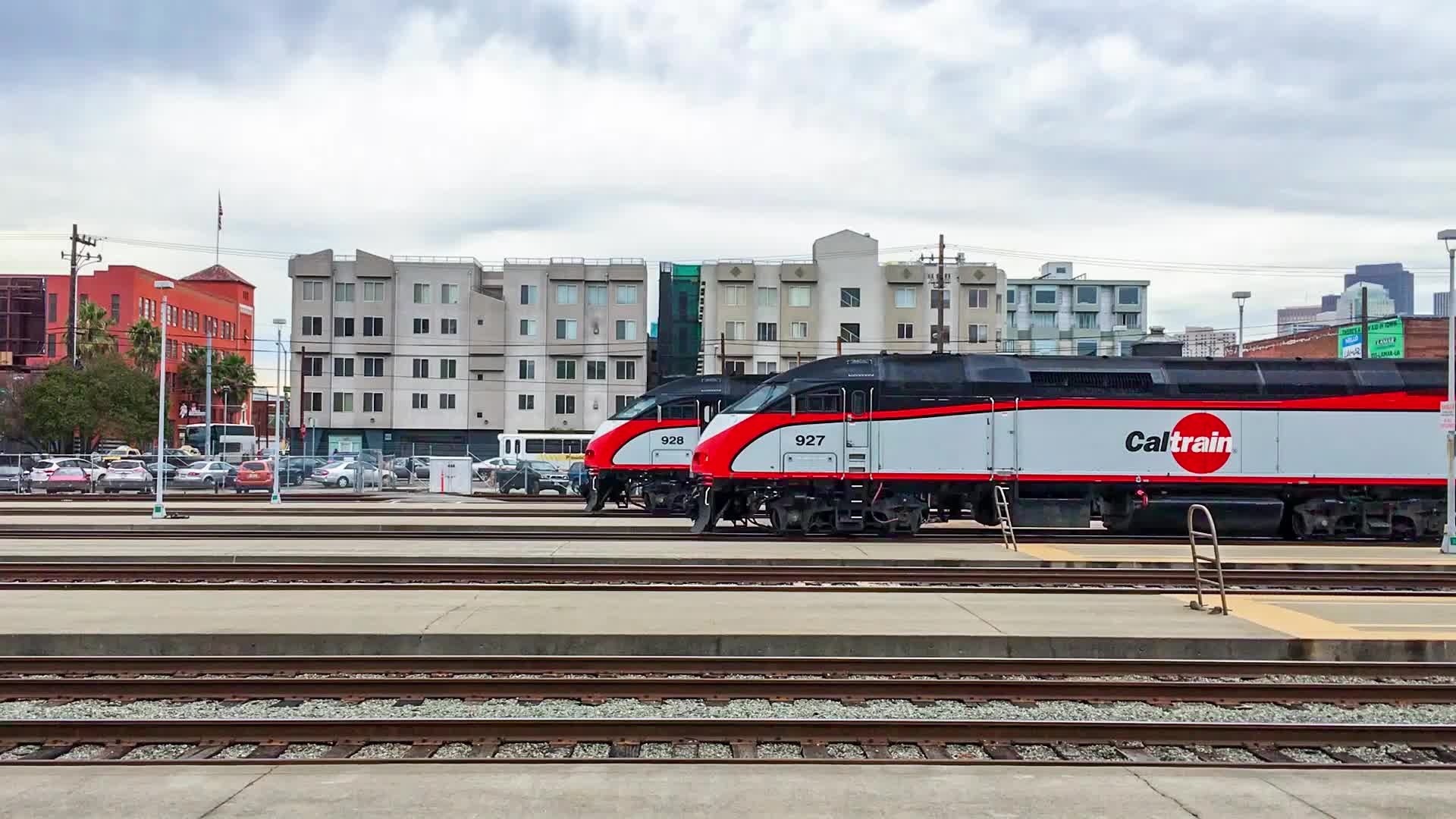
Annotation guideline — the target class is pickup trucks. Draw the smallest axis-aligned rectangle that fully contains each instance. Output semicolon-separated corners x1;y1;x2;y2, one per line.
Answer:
496;459;570;496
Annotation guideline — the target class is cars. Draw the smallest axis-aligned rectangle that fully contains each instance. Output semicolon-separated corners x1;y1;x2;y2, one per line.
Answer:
310;461;398;488
234;460;280;494
567;462;589;496
384;457;430;478
223;452;385;488
172;461;236;490
0;446;205;494
472;457;519;481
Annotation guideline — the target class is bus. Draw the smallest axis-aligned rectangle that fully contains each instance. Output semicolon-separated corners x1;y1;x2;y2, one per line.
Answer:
497;433;594;473
183;423;257;463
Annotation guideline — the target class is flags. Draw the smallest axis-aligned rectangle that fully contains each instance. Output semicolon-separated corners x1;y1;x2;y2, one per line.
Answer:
218;197;223;230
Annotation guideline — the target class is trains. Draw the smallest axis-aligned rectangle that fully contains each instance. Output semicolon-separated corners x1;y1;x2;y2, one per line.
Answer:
687;354;1456;537
581;370;786;515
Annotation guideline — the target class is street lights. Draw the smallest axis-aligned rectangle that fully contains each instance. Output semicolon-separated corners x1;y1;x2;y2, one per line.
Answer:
152;281;176;520
270;318;287;505
222;385;232;490
1436;227;1456;556
1231;290;1253;356
